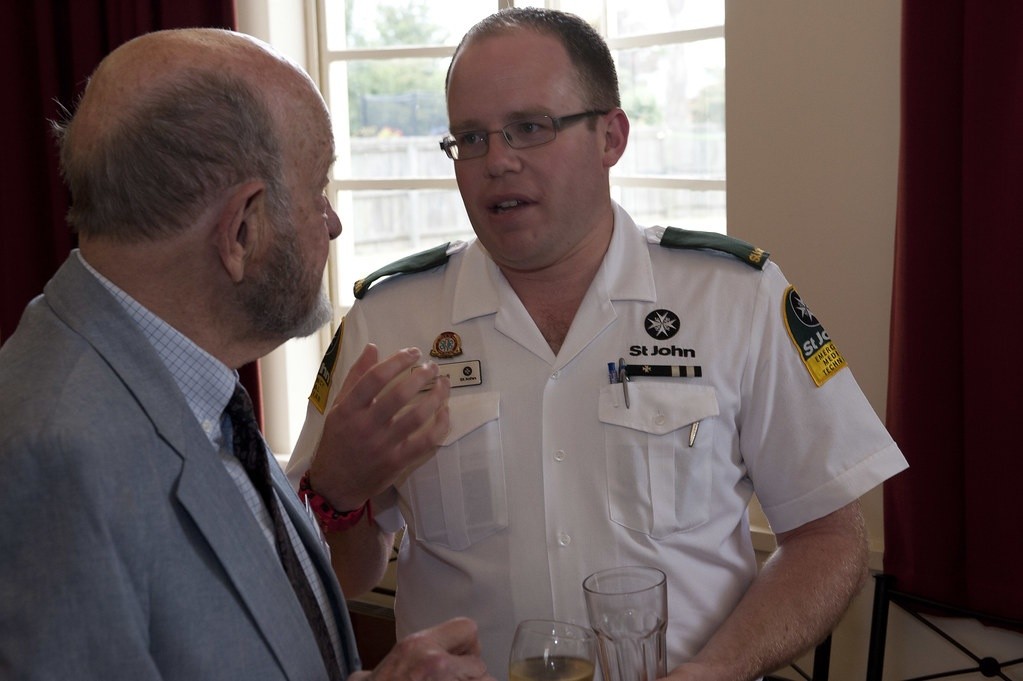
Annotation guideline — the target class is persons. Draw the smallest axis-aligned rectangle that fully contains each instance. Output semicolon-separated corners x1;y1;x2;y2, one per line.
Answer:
284;8;909;680
0;29;491;680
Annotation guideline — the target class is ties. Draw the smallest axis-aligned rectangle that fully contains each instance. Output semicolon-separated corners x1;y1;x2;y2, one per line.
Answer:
224;382;343;680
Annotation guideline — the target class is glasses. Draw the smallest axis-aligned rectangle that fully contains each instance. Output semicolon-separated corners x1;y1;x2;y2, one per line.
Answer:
439;110;606;162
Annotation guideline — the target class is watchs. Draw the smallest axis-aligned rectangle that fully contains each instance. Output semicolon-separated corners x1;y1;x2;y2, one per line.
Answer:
298;469;374;535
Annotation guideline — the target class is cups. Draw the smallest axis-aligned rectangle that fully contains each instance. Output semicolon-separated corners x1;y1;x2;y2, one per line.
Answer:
583;565;668;681
506;619;596;681
